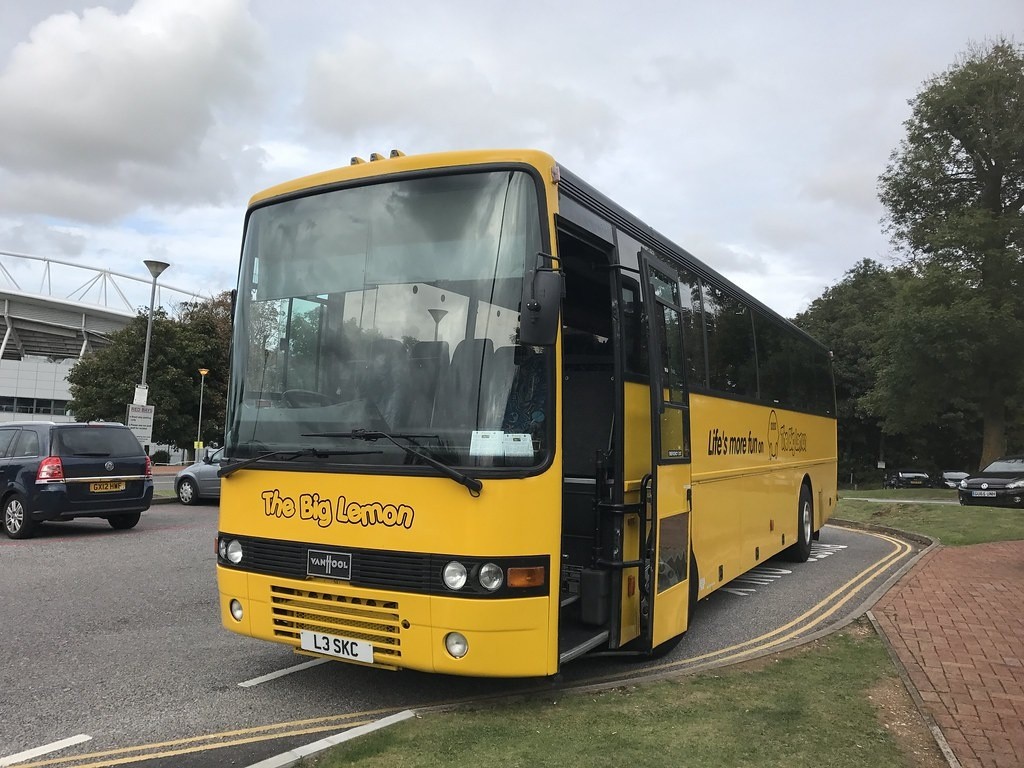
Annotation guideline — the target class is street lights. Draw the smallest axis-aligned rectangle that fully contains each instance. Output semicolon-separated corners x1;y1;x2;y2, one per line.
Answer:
427;309;448;340
142;260;170;387
196;368;209;462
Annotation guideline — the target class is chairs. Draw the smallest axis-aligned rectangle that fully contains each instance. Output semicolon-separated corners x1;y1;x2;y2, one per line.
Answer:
355;333;541;439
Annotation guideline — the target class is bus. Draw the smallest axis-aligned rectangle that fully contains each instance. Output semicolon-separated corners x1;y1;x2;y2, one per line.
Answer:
215;146;838;679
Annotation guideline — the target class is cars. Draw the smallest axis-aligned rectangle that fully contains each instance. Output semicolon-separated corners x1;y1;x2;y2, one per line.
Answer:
937;471;971;489
884;472;933;489
959;455;1024;509
173;447;221;505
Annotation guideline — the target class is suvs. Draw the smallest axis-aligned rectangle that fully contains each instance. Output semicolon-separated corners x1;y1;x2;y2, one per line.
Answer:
0;421;154;539
